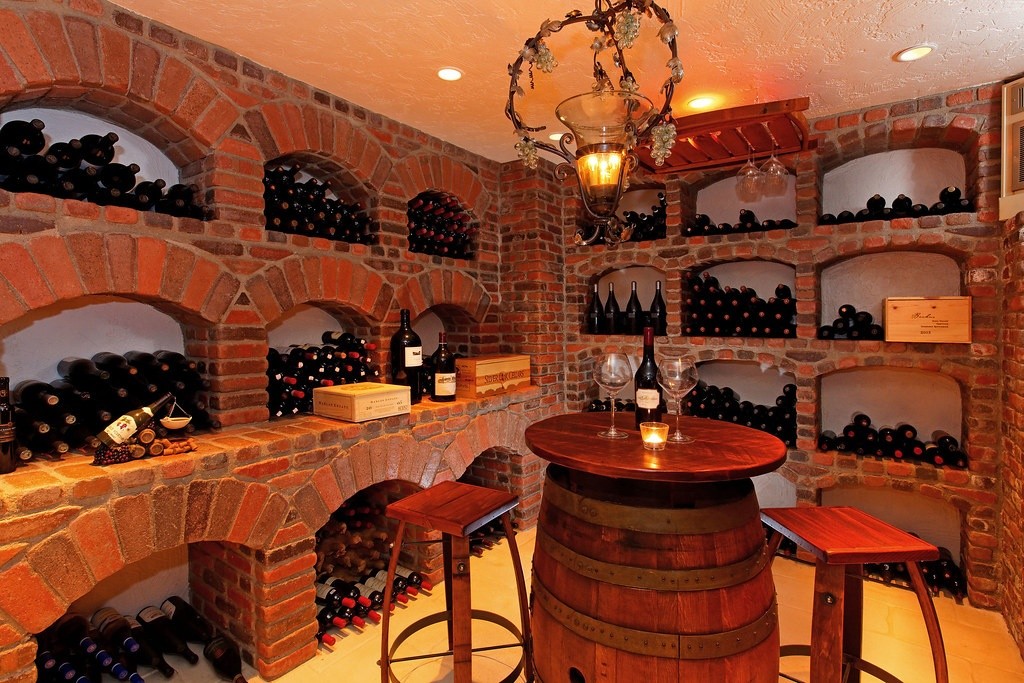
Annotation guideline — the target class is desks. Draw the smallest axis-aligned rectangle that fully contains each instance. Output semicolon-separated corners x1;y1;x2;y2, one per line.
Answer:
525;412;787;683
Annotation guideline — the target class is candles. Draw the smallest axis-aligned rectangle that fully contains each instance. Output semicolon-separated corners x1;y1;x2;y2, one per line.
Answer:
639;421;670;452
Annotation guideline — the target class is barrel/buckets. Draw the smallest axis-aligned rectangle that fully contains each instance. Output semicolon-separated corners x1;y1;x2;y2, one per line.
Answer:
526;463;780;683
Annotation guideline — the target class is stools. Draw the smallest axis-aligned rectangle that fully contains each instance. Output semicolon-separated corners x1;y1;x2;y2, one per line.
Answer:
760;505;949;683
378;477;530;683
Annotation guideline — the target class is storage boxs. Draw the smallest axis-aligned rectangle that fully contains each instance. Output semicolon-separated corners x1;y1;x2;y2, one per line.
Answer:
311;381;411;426
883;295;972;342
455;352;531;401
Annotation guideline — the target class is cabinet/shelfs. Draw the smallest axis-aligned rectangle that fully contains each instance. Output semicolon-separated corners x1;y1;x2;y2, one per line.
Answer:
997;75;1024;221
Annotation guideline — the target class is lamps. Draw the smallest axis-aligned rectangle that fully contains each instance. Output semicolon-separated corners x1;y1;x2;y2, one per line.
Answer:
504;0;684;249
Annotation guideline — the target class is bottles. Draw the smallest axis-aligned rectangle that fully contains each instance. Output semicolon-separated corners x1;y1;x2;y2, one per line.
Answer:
468;518;519;554
36;595;248;683
818;187;973;223
635;328;663;431
0;350;221;475
588;397;666;414
865;532;966;596
267;330;382;419
818;412;968;469
316;503;434;645
391;308;464;405
684;377;797;448
585;193;665;243
819;305;884;340
0;119;214;220
761;522;796;557
260;163;375;244
409;195;477;258
685;271;798;339
686;208;797;234
586;280;667;335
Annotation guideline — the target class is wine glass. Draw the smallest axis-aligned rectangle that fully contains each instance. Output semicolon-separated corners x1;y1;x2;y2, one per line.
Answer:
657;358;699;444
736;138;787;202
591;352;633;440
